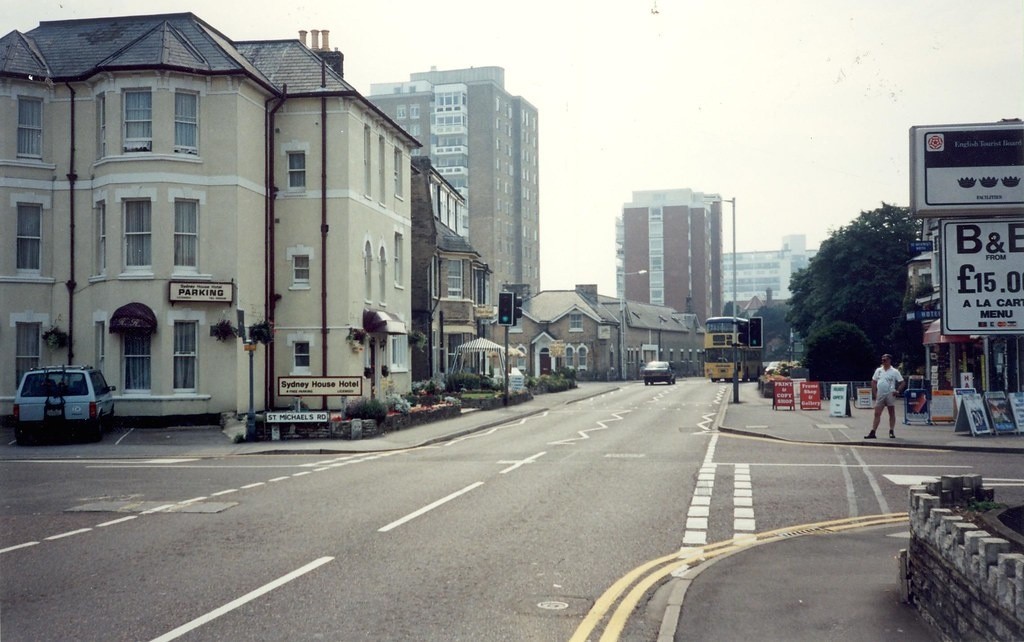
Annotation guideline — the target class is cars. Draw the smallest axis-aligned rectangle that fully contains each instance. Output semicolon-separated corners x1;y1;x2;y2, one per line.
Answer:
763;360;785;375
788;360;802;370
644;361;675;384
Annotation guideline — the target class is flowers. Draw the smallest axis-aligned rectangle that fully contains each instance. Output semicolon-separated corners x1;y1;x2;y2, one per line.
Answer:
210;312;233;343
758;374;791;383
407;329;428;352
249;319;279;345
41;313;68;353
346;328;368;354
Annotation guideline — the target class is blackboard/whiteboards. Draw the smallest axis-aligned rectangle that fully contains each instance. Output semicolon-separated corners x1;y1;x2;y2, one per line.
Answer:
909;376;931;399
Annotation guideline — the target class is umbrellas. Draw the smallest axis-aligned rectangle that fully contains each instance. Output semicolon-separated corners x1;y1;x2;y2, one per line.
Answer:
486;342;526;374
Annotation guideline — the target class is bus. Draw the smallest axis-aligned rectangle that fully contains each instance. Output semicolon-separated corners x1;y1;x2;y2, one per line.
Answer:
704;316;762;382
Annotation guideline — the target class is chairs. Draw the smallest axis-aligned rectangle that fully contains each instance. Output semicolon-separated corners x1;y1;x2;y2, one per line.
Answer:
42;379;57;391
72;380;84;392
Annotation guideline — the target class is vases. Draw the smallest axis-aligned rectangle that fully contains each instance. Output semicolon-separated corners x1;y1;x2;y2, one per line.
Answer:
249;326;267;338
408;336;423;349
353;334;366;340
763;382;774;398
208;325;219;337
759;380;764;394
758;379;761;390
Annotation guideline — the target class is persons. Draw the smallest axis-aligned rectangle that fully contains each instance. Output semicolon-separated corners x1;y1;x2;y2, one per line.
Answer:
864;353;906;440
640;359;647;379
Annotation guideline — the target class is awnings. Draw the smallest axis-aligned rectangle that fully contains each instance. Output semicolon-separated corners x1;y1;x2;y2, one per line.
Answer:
365;308;409;337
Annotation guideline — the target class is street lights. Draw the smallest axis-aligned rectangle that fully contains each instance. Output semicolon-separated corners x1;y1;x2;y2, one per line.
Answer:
703;197;739;404
622;269;648;382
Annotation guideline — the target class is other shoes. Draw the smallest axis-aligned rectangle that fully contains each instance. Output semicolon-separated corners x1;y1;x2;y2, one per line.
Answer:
889;432;895;438
864;433;876;439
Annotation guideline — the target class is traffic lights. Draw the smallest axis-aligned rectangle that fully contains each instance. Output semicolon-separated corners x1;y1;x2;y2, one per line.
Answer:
515;295;524;324
497;293;514;325
738;317;762;348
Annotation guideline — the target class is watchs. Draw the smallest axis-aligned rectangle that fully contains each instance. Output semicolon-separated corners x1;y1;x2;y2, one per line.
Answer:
896;390;901;395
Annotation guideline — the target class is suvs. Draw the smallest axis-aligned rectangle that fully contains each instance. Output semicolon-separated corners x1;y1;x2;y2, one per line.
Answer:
13;365;118;441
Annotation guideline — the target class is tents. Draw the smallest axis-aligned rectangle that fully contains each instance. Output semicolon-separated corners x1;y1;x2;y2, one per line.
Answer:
453;336;505;379
